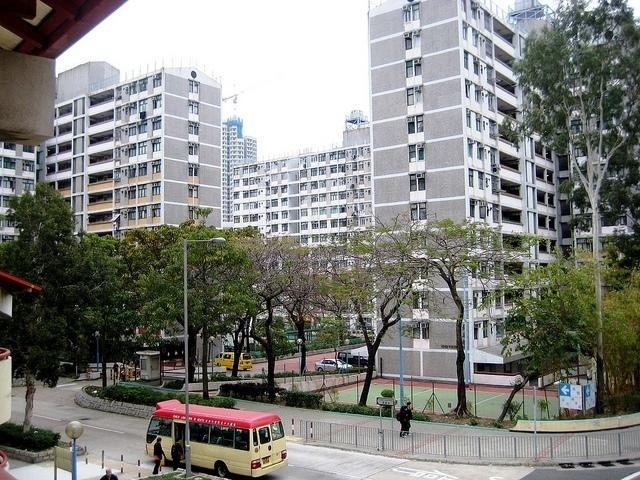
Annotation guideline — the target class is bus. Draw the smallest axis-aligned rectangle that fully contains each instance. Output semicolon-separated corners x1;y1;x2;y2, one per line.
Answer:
350;328;376;338
142;396;291;480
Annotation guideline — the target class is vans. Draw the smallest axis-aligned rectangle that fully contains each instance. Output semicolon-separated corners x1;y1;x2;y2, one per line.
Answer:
213;351;252;371
335;350;368;369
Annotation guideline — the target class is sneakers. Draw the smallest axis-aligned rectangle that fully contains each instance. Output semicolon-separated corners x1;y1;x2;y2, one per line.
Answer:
402;433;411;438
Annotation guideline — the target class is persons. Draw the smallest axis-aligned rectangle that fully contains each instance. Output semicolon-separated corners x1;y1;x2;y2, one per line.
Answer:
99;468;120;480
404;401;413;435
152;436;167;475
170;437;184;471
398;406;409;437
111;360;120;380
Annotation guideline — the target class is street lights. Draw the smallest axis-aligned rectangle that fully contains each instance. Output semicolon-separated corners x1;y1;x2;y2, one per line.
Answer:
95;330;102;373
344;339;350;369
296;338;305;378
182;237;228;479
64;419;86;480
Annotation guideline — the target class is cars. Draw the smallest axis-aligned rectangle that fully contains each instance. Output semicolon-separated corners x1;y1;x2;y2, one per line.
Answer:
315;358;353;373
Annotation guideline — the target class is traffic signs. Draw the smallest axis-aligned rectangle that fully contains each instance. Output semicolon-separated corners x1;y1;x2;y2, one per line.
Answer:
377;396;395;407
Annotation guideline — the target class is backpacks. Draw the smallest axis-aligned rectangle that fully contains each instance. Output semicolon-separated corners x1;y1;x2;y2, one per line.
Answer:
170;444;180;460
396;412;402;421
